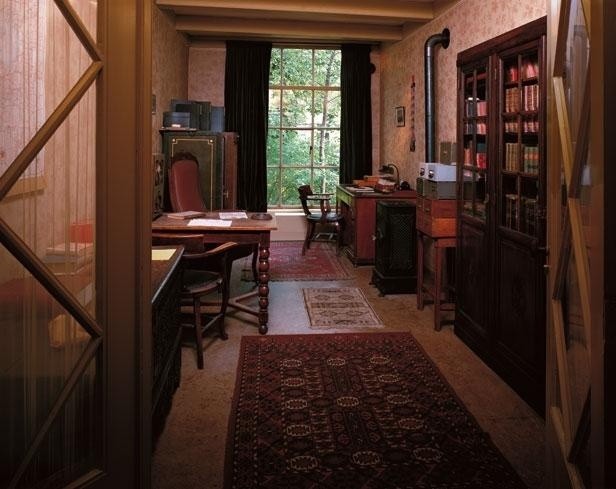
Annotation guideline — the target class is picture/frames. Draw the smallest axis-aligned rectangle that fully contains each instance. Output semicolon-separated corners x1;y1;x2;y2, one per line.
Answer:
395;106;405;127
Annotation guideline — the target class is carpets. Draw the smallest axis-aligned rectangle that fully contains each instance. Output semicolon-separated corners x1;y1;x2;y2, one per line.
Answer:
302;287;382;328
239;239;355;281
221;331;529;488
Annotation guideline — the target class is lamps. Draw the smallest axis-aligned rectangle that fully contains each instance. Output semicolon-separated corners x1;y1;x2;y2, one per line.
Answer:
378;163;399;185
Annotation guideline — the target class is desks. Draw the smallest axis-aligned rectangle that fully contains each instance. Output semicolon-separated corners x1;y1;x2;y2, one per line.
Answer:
336;183;417;267
151;210;278;334
417;231;456;331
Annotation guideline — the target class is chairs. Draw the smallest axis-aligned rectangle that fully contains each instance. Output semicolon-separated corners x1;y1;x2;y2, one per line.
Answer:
151;232;239;369
167;152;211;212
298;184;344;256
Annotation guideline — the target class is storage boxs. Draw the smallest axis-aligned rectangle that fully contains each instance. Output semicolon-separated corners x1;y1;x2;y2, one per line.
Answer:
163;101;210;129
439;142;457;164
416;163;456;238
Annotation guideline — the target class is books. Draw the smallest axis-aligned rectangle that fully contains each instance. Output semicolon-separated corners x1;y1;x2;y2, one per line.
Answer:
346;186;374;193
36;221;94;275
454;51;539;240
363;175;381;182
167;210;204;219
352;180;376;187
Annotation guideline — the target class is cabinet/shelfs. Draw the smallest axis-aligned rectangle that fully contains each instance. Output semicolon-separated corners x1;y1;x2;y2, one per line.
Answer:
454;14;546;419
0;245;184;488
368;199;416;297
163;130;225;212
